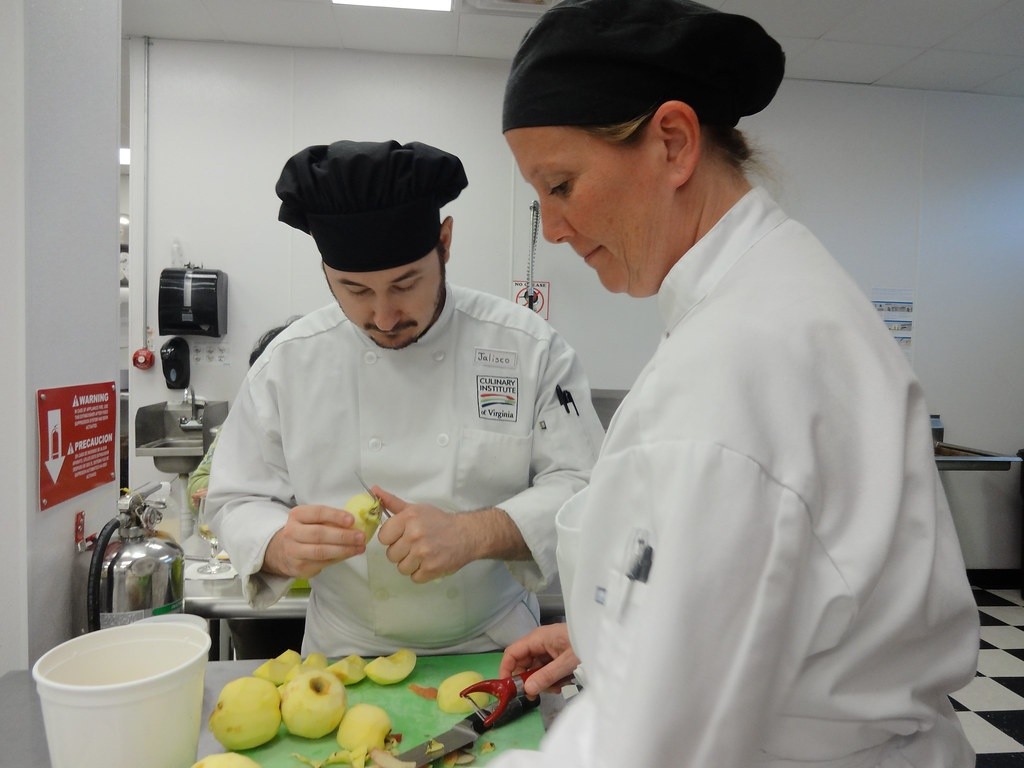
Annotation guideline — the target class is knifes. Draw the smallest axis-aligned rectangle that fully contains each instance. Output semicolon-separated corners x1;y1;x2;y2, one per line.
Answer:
393;691;541;768
355;471;392;518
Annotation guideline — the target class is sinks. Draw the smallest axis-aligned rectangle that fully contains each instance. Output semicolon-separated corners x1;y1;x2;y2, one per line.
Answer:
135;438;204;473
934;440;1023;472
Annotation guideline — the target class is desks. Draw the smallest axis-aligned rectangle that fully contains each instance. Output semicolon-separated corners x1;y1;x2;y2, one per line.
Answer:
1;645;579;767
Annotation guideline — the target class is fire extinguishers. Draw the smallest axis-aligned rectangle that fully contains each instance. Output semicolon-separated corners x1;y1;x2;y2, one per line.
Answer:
85;477;183;635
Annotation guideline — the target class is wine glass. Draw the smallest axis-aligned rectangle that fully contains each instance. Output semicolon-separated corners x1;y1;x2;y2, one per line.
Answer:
196;496;231;575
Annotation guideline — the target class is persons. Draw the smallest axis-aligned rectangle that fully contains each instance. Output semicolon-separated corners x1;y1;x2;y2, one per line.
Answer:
186;316;303;516
203;140;606;662
368;0;979;768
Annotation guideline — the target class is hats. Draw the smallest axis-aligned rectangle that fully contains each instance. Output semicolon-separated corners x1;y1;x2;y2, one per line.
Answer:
502;0;785;135
276;139;468;272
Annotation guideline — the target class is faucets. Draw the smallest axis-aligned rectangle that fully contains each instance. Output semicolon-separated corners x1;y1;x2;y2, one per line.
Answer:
179;385;204;430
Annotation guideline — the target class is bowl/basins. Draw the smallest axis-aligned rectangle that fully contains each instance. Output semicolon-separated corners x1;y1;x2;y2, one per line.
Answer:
31;621;212;768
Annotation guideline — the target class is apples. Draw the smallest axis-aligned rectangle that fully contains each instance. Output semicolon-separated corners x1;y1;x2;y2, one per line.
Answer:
342;491;381;546
190;649;492;768
